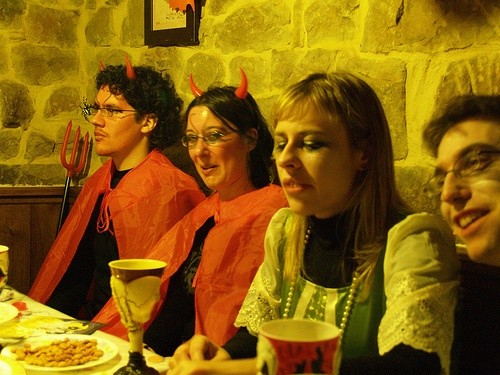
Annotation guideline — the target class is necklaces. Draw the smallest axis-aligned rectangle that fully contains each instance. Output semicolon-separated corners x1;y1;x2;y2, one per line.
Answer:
283;226;359;343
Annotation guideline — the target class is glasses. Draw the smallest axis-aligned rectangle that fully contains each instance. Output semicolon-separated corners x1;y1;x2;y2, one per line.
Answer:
422;150;500;200
179;130;240;148
84;106;138;116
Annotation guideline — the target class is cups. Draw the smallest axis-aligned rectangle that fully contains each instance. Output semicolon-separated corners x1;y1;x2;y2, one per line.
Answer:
255;318;342;375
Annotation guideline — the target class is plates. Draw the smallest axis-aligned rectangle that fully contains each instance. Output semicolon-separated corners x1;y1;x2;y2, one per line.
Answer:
0;333;120;372
0;316;107;347
0;355;27;375
0;302;18;325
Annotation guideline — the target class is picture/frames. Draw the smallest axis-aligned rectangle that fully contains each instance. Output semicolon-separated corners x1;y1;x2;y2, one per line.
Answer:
143;0;200;50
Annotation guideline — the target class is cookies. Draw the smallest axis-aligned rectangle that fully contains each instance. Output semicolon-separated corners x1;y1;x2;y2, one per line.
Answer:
11;337;104;366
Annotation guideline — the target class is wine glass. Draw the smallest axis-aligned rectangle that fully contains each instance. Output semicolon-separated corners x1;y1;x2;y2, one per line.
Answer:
108;259;168;375
0;245;14;302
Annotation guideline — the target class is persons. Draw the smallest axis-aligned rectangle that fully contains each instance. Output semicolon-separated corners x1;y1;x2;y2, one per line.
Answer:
90;67;291;359
26;52;207;322
421;92;500;375
165;70;459;375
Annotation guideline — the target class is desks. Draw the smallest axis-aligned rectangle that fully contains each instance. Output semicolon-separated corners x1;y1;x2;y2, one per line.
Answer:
0;281;176;375
